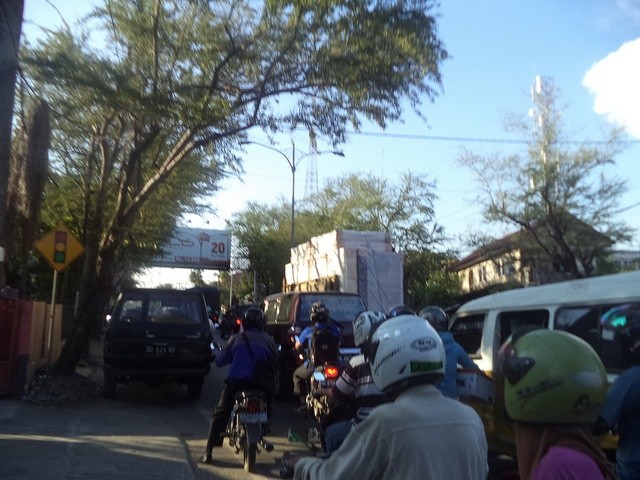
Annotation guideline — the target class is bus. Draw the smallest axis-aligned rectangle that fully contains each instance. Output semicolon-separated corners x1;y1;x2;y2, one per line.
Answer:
192;287;218;324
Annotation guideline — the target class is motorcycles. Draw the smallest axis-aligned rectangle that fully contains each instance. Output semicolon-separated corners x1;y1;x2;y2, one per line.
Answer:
288;324;343;426
210;342;282;472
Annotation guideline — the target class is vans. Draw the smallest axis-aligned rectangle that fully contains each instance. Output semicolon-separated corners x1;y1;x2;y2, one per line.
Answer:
264;292;370;369
447;271;639;383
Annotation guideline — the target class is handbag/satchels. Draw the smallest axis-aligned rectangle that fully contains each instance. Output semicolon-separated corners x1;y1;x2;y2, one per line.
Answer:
255;359;280;396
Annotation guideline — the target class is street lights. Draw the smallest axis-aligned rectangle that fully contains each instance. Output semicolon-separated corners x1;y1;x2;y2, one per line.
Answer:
238;139;345;247
176;231;202;285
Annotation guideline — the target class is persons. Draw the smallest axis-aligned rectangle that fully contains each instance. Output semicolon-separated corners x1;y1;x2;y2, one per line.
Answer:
497;324;618;480
313;310;395;451
293;306;345;413
199;306;280;464
593;301;640;480
418;305;480;401
280;313;490;480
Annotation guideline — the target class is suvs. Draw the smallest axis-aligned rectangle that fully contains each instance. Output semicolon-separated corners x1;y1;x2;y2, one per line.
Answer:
103;289;216;398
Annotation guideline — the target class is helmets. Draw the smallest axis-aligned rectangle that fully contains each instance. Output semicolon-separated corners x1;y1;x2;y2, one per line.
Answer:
352;310;387;347
497;323;608;425
389;306;417;318
418;305;449;332
599;303;640;367
363;314;447;392
309;301;329;322
242;309;264;329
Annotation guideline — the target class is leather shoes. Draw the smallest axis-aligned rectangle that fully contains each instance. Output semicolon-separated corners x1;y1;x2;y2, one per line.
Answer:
200;453;212;464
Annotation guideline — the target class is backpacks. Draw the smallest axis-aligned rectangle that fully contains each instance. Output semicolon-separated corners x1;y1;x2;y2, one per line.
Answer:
310;324;339;366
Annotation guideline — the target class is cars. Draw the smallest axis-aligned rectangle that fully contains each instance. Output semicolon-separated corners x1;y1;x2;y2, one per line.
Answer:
219;305;264;339
